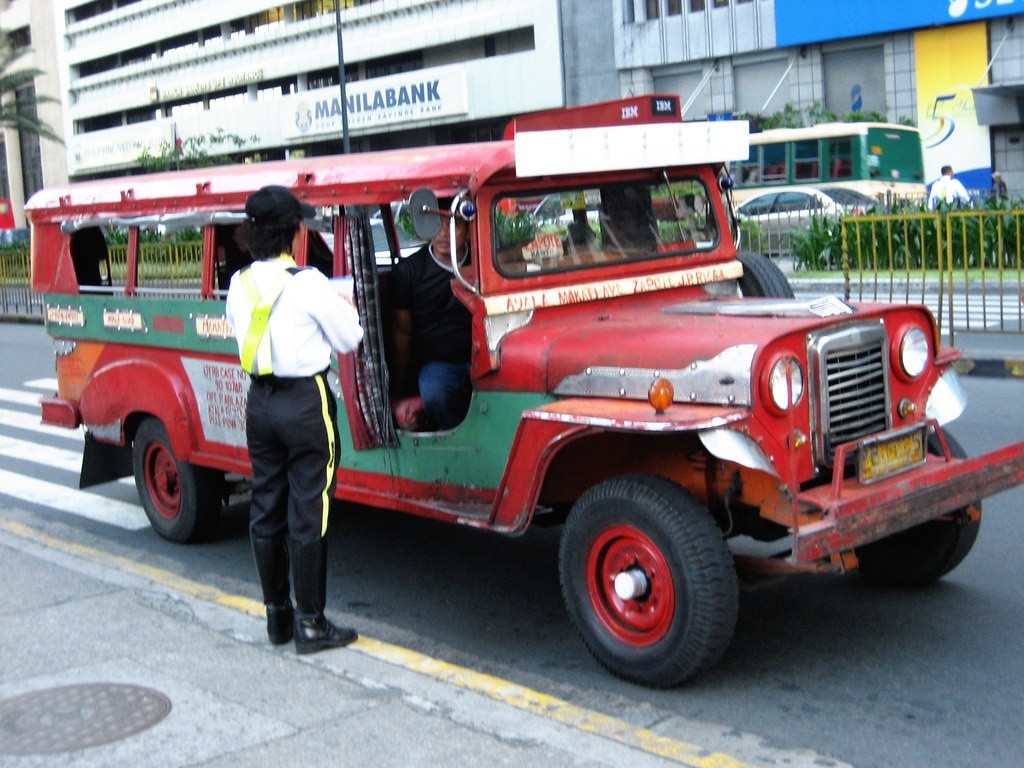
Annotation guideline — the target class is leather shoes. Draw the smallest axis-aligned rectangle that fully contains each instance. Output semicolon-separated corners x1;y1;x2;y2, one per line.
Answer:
294;613;358;654
266;599;296;645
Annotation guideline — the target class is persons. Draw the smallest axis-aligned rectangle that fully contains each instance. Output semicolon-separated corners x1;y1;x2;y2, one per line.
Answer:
386;201;472;430
926;166;1009;212
225;188;358;657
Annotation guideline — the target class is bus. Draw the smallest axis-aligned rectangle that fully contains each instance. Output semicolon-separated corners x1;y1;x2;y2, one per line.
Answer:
716;120;926;186
21;92;1024;691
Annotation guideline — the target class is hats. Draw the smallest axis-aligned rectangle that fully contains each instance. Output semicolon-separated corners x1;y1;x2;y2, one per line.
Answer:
991;171;1002;178
245;186;316;221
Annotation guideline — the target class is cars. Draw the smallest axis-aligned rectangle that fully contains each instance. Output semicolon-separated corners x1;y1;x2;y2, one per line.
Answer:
732;181;891;254
524;186;602;231
